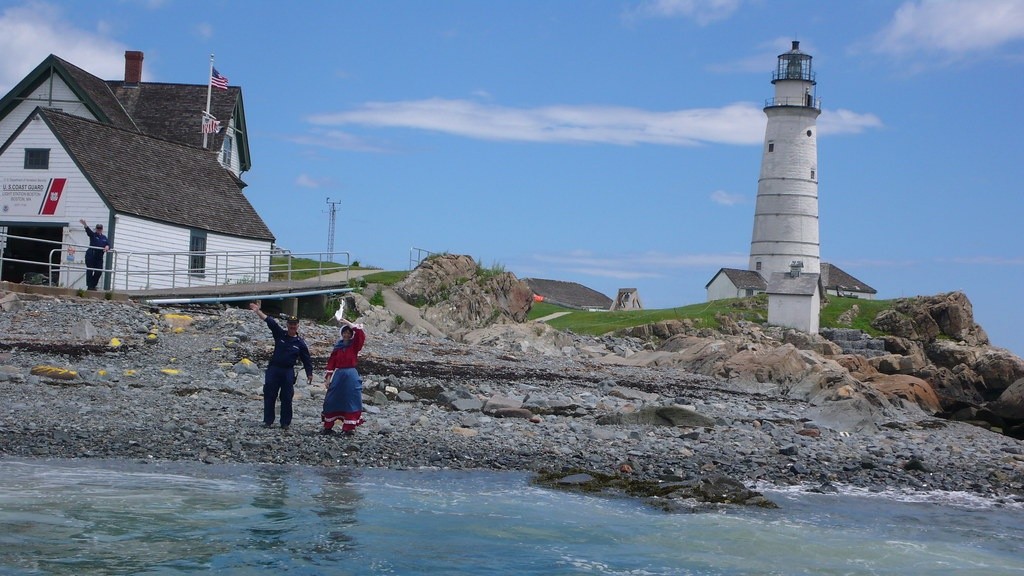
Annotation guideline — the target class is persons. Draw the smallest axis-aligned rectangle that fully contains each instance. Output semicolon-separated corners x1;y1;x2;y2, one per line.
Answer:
250;303;313;432
321;318;365;436
80;219;110;291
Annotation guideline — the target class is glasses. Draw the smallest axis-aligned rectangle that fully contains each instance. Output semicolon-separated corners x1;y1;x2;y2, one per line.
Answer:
96;227;103;229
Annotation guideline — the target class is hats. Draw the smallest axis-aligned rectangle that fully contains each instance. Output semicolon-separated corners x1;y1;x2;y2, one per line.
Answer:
287;315;299;324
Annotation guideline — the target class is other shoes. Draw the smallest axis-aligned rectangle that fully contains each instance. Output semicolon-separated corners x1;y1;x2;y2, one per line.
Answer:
281;425;290;429
264;423;271;428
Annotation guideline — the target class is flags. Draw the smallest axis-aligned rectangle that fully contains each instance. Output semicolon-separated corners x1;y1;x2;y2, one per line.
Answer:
211;66;229;89
201;116;219;133
